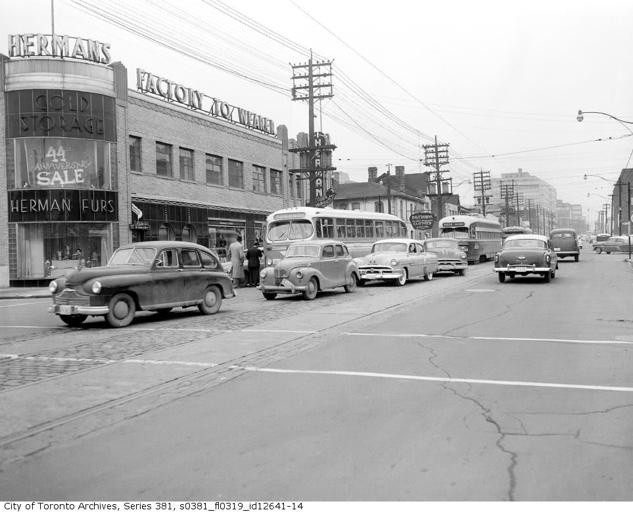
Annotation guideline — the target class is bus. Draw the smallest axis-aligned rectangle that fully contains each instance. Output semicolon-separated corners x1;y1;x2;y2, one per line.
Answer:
436;213;502;265
259;206;409;259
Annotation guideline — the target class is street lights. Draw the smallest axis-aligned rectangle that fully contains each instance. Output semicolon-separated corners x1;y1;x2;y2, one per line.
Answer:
583;174;623;236
576;110;632;136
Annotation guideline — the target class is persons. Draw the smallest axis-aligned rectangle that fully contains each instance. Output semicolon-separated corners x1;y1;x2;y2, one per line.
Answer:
225;235;245;289
245;240;262;287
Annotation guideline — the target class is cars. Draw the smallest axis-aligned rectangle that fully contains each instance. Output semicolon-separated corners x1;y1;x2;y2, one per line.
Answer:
46;239;236;328
255;239;361;300
494;225;632;283
351;239;439;286
423;238;469;276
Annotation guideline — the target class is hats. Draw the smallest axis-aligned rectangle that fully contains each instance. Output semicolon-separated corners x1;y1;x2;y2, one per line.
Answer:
251;240;260;246
